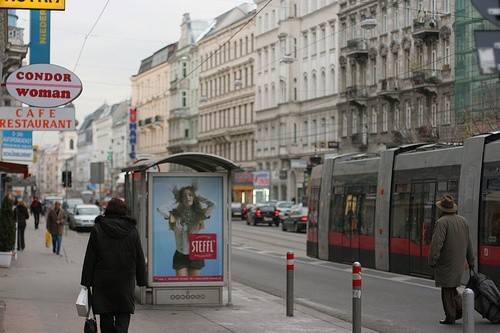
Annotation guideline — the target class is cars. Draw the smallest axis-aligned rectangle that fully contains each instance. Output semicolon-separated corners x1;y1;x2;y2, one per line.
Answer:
282;206;308;234
43;195;64;214
67;204;101;231
61;199;84;221
231;202;247;221
246;204;279;227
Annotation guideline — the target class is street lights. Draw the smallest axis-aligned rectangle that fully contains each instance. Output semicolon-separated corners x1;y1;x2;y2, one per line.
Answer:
60;157;75;235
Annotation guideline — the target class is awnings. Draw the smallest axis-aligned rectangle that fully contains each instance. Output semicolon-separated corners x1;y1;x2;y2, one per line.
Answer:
0;160;29;179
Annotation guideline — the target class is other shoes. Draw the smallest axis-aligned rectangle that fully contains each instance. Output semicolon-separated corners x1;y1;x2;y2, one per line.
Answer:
53;250;56;253
57;252;59;255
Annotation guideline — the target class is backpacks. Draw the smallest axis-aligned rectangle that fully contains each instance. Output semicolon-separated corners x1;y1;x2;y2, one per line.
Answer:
465;266;500;323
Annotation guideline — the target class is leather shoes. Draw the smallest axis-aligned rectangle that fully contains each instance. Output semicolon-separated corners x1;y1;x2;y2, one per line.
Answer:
440;320;454;324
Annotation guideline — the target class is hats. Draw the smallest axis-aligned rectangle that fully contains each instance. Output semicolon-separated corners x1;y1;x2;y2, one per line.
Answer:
436;194;457;213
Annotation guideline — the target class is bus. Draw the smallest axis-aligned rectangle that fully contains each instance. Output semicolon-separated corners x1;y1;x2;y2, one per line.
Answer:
304;131;500;289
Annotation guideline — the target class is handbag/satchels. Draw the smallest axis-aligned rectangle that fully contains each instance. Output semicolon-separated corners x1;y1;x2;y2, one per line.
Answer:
75;288;89;317
46;232;50;247
84;318;97;333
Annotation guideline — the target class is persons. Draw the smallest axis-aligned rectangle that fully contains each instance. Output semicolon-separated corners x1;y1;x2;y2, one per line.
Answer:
428;194;475;325
347;206;353;227
90;190;124;215
13;195;67;255
157;181;216;276
81;198;149;333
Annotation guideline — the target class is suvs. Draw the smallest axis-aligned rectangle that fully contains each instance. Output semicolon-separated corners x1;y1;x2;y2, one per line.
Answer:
268;200;295;221
